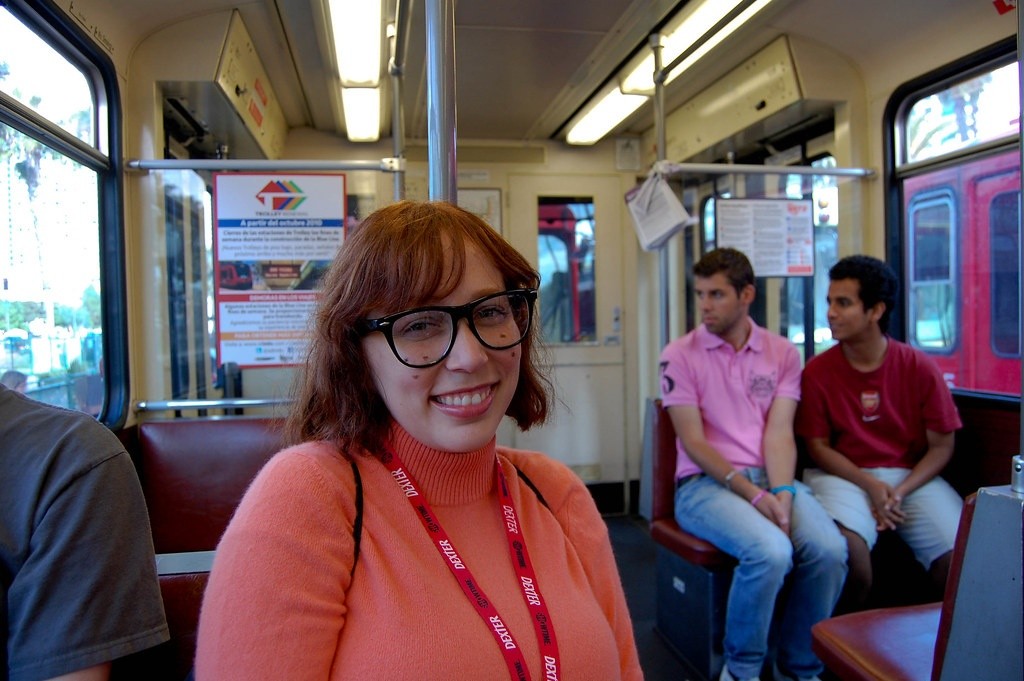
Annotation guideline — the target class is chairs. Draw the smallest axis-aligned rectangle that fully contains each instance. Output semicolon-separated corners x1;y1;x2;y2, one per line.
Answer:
811;486;1024;681
648;396;823;678
111;414;329;680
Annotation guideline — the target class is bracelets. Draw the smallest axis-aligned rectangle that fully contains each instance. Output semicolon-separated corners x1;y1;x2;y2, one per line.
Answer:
722;468;740;490
773;485;797;500
750;489;769;508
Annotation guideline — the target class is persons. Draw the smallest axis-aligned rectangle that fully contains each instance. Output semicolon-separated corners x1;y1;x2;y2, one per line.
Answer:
68;375;103;418
660;248;850;681
209;355;218;383
0;369;29;398
795;254;972;612
0;372;171;681
192;198;649;681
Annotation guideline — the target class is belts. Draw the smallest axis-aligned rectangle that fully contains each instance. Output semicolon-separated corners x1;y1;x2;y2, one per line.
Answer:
676;472;708;489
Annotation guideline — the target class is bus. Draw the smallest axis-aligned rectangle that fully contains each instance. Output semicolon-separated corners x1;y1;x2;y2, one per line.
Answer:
219;263;252;290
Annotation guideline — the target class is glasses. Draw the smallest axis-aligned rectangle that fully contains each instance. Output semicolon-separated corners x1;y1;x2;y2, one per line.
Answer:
357;286;538;368
